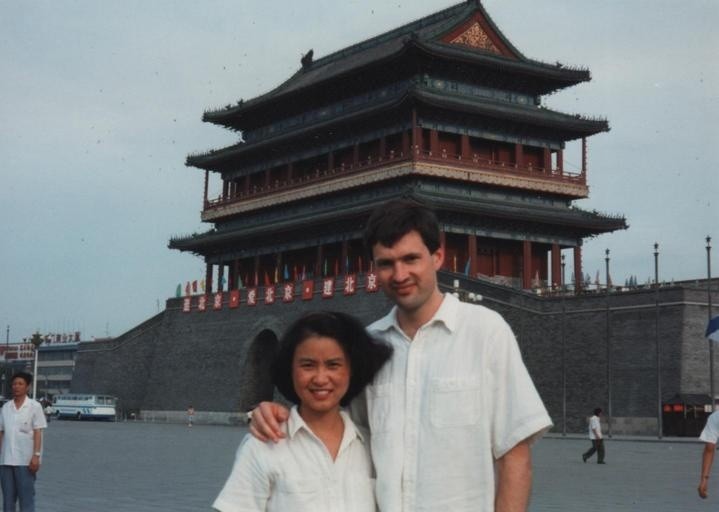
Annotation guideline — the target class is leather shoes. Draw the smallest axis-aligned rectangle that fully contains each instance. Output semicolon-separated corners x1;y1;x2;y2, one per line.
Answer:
597;460;606;464
582;454;587;463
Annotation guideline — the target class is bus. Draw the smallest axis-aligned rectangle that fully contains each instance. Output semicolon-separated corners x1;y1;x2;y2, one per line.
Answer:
51;393;116;420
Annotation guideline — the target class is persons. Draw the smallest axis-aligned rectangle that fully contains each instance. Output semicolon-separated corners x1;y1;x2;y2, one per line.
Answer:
697;409;719;499
0;371;48;512
210;308;393;511
246;409;253;423
45;404;53;423
582;406;606;464
248;196;553;511
187;405;196;427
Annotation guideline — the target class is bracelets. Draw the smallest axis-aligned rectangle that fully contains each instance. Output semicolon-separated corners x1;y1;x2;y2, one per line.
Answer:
33;452;41;456
701;474;708;479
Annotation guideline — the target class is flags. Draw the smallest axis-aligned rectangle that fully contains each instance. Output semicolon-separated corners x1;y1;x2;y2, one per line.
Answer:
464;255;472;275
175;254;374;299
453;255;458;272
